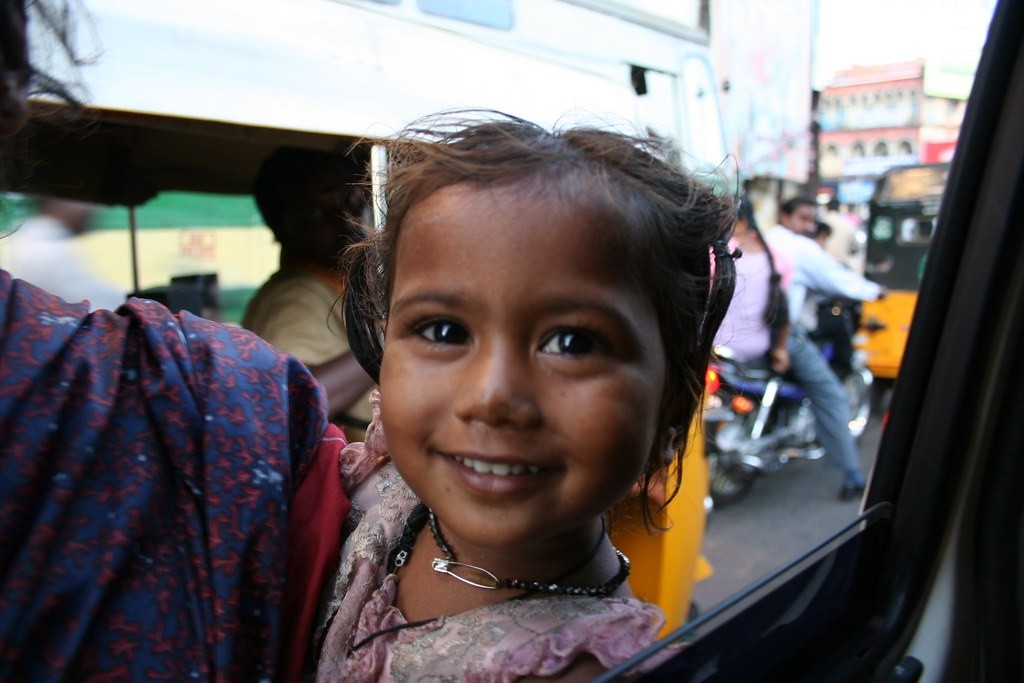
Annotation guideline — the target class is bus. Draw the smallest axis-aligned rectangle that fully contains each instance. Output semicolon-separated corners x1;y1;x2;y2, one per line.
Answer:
0;1;738;337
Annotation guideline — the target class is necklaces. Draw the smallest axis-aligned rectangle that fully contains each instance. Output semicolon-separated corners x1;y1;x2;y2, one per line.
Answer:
426;506;631;598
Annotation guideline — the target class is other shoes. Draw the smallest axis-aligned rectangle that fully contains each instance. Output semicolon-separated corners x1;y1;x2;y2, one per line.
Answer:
838;478;866;500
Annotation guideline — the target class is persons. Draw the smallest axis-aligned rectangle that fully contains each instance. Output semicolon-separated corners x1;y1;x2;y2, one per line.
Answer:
0;0;350;683
315;106;743;683
242;142;387;444
0;191;126;314
709;198;888;500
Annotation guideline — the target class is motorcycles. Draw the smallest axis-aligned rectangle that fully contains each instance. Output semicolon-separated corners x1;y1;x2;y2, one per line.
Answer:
850;161;951;419
701;292;885;509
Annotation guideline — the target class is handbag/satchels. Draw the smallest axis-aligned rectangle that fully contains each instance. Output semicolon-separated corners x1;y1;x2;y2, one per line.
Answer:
761;235;787;328
